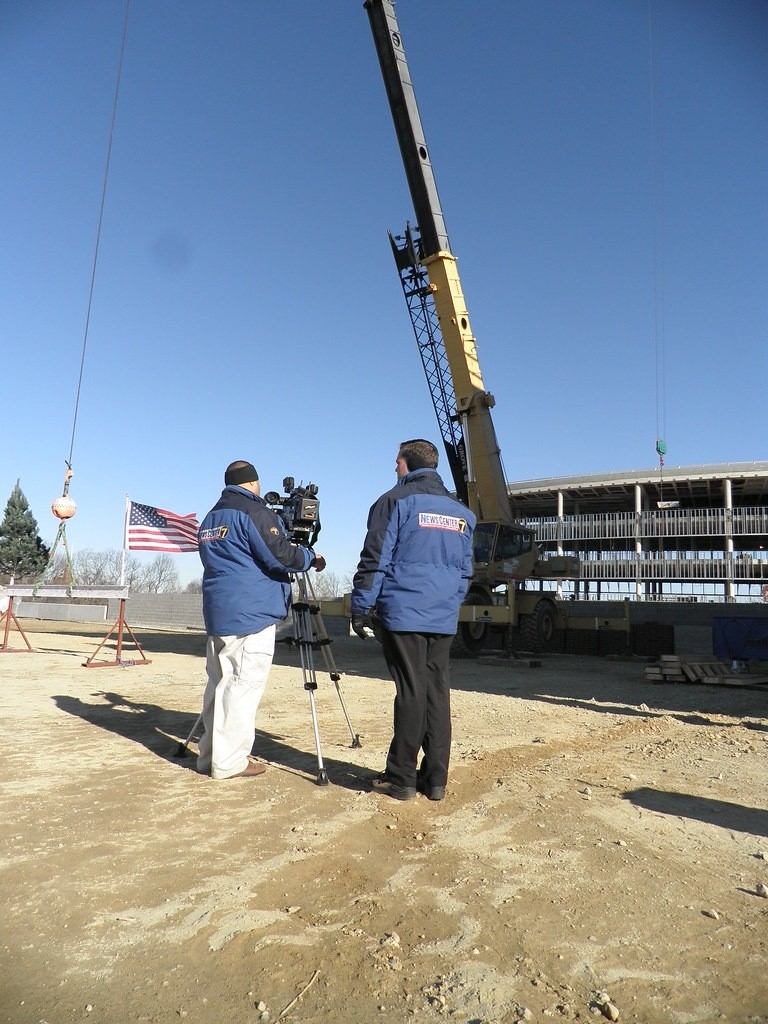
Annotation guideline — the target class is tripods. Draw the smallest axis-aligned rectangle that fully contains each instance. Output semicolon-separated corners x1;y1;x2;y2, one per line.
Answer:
173;570;363;787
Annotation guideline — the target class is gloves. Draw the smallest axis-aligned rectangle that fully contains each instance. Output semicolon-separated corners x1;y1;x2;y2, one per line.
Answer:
350;610;376;641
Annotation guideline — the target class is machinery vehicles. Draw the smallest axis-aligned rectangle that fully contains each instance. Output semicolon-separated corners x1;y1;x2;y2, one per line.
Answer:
361;1;639;658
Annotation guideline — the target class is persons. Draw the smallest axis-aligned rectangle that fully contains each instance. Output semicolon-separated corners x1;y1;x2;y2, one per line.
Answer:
197;462;326;779
350;440;477;799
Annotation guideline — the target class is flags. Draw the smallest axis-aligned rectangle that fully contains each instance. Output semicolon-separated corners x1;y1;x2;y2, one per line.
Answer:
125;500;201;552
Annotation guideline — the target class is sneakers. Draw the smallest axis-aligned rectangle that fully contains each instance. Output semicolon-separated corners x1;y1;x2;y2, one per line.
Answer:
416;770;446;800
223;758;266;779
372;770;417;800
